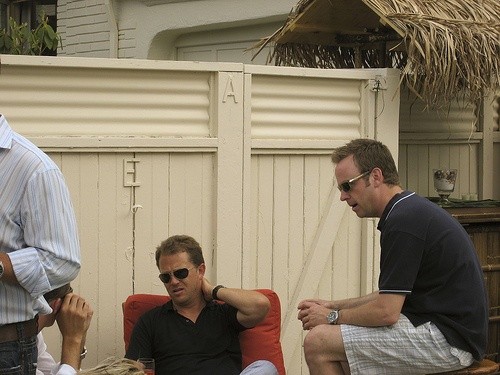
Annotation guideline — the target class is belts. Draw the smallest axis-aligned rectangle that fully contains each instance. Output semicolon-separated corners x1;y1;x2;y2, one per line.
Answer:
0;318;38;343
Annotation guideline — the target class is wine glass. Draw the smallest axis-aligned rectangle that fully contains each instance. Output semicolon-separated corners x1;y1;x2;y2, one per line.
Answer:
434;168;457;207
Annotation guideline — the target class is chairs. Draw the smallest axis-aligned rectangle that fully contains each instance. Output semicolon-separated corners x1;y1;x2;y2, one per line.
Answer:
121;289;287;375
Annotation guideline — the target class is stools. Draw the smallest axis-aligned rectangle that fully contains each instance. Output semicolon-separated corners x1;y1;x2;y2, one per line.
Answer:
427;358;500;375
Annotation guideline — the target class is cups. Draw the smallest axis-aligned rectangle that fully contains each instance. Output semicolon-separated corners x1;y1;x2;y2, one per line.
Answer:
137;358;155;375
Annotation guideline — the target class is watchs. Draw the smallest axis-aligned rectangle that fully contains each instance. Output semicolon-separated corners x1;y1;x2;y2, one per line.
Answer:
0;261;5;278
327;308;342;325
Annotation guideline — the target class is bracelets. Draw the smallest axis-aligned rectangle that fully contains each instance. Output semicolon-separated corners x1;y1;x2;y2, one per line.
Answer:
212;285;224;301
80;346;88;360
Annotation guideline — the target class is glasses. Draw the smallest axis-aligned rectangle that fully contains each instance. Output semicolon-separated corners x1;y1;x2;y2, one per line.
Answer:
159;267;197;283
336;171;371;192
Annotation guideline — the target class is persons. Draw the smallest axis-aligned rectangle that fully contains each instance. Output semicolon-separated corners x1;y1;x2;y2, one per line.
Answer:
0;115;80;374
296;139;488;375
124;236;278;375
36;283;93;375
